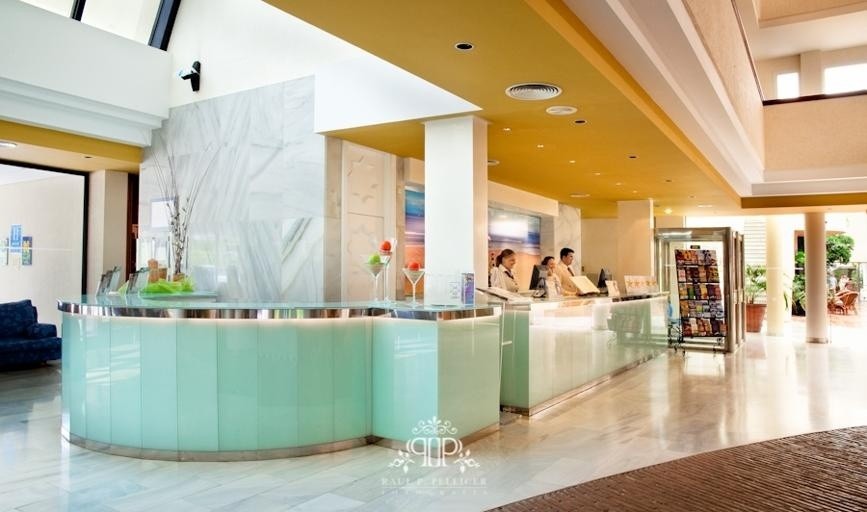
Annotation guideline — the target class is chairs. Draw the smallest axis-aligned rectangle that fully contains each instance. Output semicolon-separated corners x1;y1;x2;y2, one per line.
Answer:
828;290;858;316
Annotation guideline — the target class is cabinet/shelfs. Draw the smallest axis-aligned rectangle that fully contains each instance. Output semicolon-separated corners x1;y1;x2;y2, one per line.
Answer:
675;250;727;345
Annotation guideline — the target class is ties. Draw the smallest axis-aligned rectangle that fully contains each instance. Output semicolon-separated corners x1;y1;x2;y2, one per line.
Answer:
568;267;575;276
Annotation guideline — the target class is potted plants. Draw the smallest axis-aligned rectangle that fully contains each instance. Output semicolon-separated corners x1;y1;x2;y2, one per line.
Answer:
745;263;767;332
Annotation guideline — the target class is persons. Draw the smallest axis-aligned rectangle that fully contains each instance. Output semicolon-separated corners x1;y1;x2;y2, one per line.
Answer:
554;249;577;292
541;255;563;294
490;249;521;293
828;274;857;293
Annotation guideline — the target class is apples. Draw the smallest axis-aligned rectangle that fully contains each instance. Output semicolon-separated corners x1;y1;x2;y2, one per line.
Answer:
368;253;379;264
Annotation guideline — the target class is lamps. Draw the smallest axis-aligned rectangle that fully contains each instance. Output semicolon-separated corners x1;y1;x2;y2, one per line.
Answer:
180;62;202;91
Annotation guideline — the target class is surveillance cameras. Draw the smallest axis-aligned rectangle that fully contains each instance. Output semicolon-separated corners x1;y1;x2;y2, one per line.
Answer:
179;68;198;81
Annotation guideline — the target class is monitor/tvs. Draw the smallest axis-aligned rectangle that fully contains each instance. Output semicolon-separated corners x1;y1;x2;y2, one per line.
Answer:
597;266;612;289
529;264;550;289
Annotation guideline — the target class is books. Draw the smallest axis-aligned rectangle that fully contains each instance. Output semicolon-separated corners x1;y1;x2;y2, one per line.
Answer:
675;249;726;336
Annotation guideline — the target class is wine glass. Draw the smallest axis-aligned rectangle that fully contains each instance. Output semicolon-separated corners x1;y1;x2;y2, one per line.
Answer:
362;239;397;307
401;268;426;307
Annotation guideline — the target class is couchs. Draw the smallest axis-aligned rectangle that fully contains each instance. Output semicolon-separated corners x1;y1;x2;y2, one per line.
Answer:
1;300;61;373
